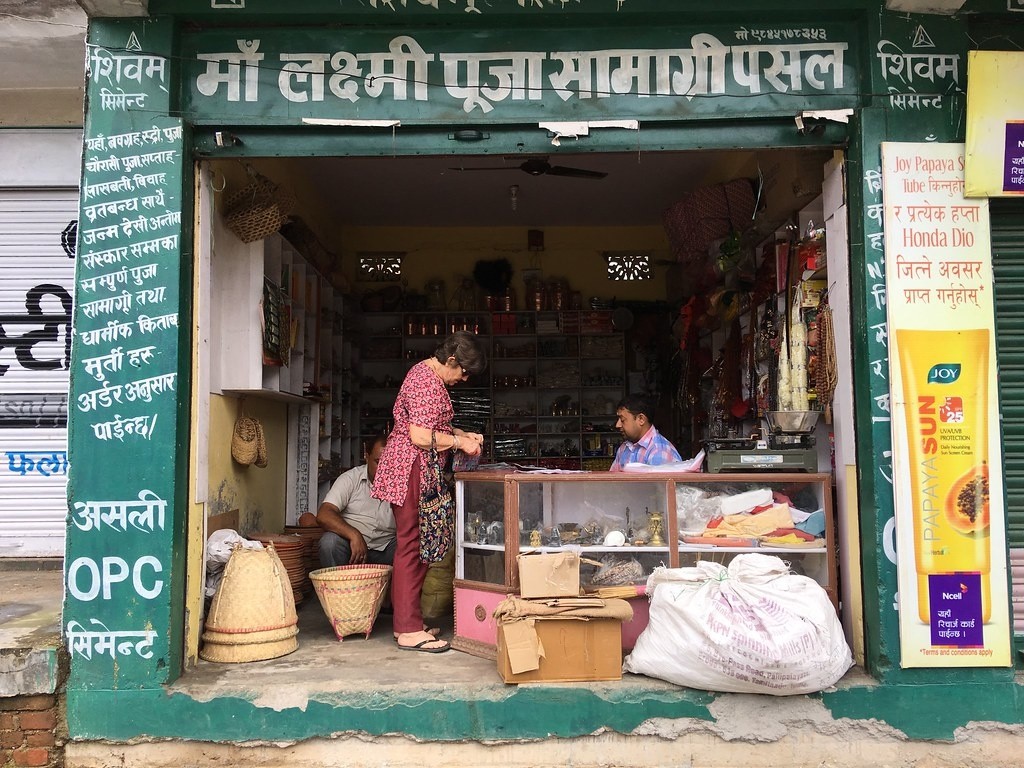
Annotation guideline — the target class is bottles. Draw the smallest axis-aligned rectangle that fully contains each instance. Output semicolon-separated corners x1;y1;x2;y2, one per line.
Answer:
473;316;481;336
406;315;416;336
551;280;565;311
483;289;498;311
460;317;469;331
419;315;429;335
450;317;458;335
500;287;514;312
532;280;545;312
432;317;441;336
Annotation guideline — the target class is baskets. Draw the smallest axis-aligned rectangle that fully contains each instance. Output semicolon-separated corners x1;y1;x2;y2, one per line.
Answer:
308;565;393;642
199;540;300;662
225;182;282;243
246;163;297;222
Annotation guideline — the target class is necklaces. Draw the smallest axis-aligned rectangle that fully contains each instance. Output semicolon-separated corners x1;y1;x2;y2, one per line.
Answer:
429;357;443;379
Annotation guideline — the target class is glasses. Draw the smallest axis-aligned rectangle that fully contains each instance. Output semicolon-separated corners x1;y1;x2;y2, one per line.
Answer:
450;354;470;377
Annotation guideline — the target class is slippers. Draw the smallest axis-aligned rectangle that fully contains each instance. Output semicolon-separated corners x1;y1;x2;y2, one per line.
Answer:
394;626;443;641
396;640;450;652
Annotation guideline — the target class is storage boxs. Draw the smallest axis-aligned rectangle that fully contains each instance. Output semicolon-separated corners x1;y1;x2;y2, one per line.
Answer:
514;545;579;602
492;594;625;686
200;543;395;665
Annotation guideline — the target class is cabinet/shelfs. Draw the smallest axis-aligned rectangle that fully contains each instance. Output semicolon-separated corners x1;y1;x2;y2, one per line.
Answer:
357;302;628;467
453;469;838;662
671;245;784;467
229;214;363;532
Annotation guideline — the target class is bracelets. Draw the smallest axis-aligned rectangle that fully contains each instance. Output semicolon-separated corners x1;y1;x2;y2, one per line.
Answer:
451;435;460;453
451;427;457;435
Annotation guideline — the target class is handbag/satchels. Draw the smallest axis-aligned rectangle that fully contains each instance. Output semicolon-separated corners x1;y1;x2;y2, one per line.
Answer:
419;481;454;563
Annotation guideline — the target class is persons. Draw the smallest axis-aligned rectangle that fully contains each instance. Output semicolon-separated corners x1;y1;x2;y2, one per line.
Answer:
607;394;683;470
313;432;401;616
370;329;485;653
